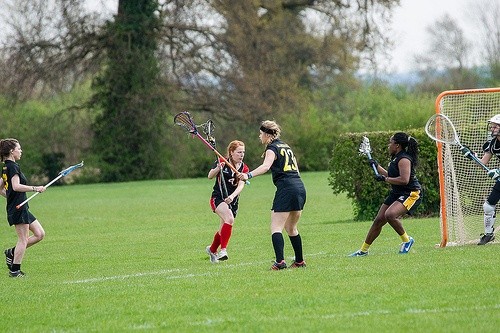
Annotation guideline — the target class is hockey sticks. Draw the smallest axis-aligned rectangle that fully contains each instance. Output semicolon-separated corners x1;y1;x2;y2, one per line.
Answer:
358;136;381;182
173;111;251;186
15;159;85;209
425;112;500;183
201;120;232;209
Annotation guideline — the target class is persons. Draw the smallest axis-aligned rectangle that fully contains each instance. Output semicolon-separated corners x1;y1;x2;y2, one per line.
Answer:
206;140;249;263
237;120;306;271
0;138;46;277
347;132;423;257
458;114;500;245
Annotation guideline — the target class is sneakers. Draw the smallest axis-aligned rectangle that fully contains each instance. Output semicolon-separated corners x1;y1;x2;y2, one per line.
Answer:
349;250;368;256
477;232;496;245
288;261;306;268
269;260;287;270
399;236;414;254
217;250;228;261
8;270;26;278
5;249;13;270
206;246;218;264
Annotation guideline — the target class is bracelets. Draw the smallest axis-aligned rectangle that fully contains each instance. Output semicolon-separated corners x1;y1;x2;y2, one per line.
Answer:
33;186;34;192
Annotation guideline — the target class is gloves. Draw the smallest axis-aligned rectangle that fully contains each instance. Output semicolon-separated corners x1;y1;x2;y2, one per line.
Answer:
459;145;477;160
368;159;378;169
486;169;500;180
374;174;386;182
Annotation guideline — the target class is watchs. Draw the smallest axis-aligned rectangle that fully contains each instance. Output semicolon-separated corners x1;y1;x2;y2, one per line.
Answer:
247;172;252;179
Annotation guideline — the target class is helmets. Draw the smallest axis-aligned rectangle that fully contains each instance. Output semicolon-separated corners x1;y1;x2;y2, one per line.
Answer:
488;114;500;125
